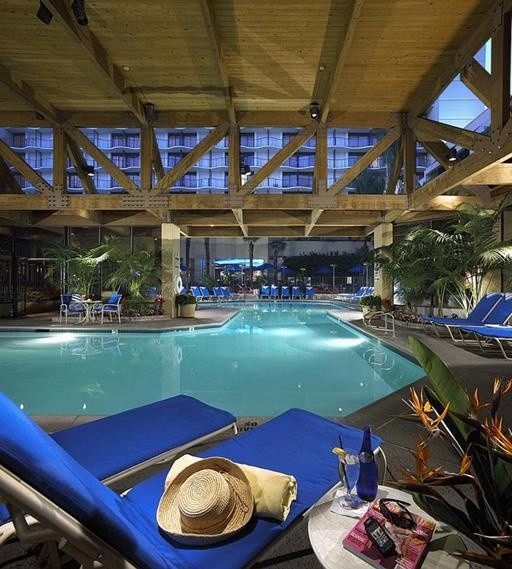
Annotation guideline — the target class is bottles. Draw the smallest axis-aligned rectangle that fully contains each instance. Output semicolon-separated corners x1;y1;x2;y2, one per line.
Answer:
357;426;379;502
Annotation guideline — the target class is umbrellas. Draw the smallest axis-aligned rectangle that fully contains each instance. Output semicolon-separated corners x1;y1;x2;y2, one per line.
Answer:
223;263;293;273
317;267;334;274
346;263;365;273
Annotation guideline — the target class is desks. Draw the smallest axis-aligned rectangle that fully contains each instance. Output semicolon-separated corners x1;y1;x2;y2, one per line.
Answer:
306;484;498;567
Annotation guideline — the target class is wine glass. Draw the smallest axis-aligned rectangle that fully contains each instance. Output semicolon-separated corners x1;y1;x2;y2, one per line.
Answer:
337;447;360;510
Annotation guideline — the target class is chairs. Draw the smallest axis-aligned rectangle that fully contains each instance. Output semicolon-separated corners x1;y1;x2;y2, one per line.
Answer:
258;285;314;299
184;281;239;304
1;392;386;569
419;288;512;362
336;285;378;309
60;293;123;325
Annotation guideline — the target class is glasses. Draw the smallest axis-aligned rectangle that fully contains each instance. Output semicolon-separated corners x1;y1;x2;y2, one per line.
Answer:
379;497;416;529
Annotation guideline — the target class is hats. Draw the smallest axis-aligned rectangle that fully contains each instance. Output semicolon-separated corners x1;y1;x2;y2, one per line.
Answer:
155;456;256;548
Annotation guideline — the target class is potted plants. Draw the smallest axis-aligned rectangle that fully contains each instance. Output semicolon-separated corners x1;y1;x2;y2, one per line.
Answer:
360;295;380;316
175;294;199;319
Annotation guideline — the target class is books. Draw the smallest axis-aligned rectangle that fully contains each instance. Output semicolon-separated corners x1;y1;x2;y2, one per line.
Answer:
341;497;436;569
